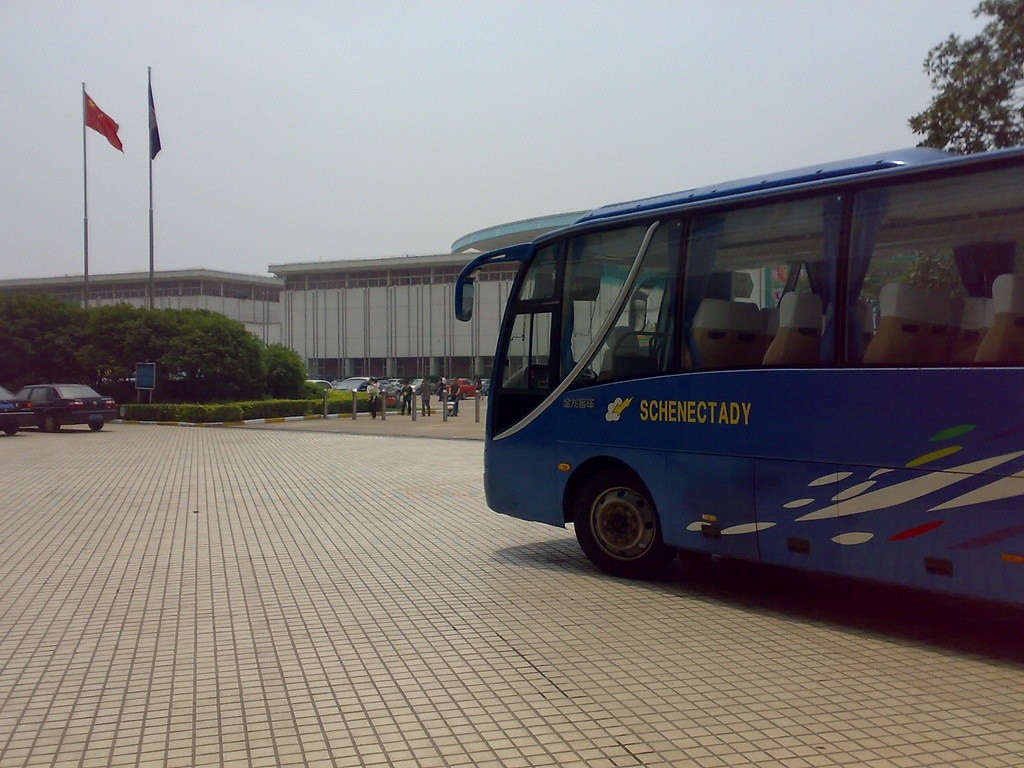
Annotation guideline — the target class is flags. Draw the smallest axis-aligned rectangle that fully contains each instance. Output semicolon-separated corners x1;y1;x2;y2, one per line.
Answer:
84;92;125;154
148;68;162;160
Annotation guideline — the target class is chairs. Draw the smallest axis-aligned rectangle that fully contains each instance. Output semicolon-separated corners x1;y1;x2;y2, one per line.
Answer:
595;273;1024;380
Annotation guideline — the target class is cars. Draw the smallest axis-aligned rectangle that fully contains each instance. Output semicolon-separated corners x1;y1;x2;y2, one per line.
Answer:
443;378;481;400
11;384;119;433
408;379;436;395
473;379;491;396
0;386;36;434
304;377;409;398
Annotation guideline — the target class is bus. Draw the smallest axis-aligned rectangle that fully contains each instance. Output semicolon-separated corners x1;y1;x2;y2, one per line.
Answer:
455;148;1024;621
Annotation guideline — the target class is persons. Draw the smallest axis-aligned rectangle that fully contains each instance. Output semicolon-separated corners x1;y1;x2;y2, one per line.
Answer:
367;377;378;419
400;381;413;416
474;375;484;400
419;377;432;417
437;375;446;401
448;376;461;417
